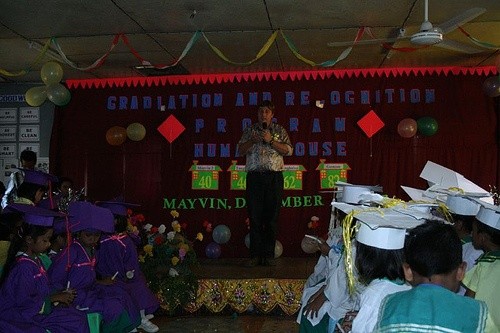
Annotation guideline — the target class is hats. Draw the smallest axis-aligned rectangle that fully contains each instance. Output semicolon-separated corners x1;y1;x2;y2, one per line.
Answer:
95;201;141;216
17;167;57;210
69;201;115;235
326;161;500;294
11;203;71;268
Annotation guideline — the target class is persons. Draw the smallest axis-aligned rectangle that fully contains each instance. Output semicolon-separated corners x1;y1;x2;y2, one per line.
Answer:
56;178;74;207
238;100;294;266
297;160;500;333
1;167;57;241
0;202;160;333
0;148;45;209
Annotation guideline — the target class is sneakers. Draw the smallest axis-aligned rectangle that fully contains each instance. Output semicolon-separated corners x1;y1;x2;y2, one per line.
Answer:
138;319;160;333
146;314;154;318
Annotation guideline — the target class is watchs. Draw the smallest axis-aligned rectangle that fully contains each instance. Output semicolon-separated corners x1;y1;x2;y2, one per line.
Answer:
269;137;278;145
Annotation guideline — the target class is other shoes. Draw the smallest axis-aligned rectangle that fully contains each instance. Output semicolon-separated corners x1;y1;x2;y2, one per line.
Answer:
264;258;278;266
243;257;257;266
130;328;138;332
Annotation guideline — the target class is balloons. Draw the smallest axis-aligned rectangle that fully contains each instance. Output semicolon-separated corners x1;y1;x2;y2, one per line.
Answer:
46;84;71;106
274;239;283;259
245;233;250;248
127;123;145;141
398;118;417;138
25;86;48;107
106;126;127;146
41;62;63;85
213;224;231;244
301;235;320;254
204;242;225;256
417;118;439;137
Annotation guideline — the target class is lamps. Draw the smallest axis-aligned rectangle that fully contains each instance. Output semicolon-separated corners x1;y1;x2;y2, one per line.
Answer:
386;26;406;60
27;41;67;66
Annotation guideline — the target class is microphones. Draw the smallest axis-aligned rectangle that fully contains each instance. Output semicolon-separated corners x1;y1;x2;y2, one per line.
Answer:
263;122;267;145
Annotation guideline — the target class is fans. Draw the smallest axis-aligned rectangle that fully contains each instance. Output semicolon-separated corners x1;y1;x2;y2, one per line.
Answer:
326;0;488;55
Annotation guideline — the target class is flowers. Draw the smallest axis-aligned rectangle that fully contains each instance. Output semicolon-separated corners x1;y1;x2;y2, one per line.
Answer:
124;204;203;313
